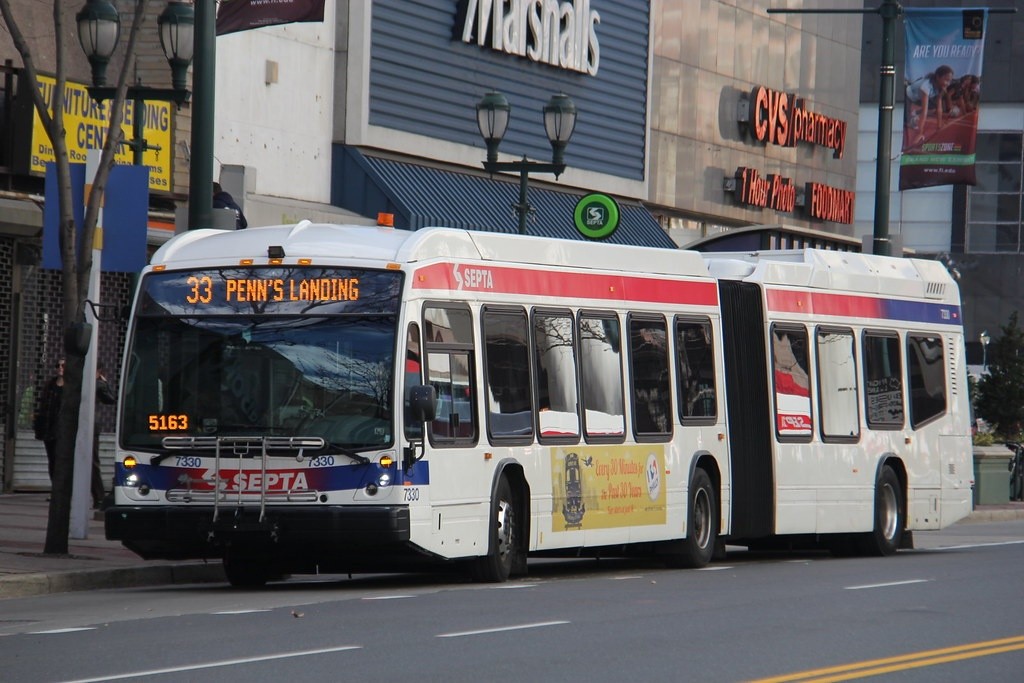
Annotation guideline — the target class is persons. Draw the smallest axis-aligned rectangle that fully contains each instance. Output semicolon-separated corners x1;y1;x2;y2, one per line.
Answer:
91;362;115;509
942;75;981;114
905;65;954;142
31;356;65;502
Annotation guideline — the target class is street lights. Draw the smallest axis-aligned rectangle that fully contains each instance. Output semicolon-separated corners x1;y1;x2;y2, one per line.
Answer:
469;85;593;238
980;329;1000;377
73;0;202;308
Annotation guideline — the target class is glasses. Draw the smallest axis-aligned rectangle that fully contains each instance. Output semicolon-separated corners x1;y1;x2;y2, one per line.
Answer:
55;364;64;368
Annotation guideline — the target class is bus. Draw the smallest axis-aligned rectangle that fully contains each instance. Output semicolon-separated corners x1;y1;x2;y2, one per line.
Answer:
102;215;975;588
